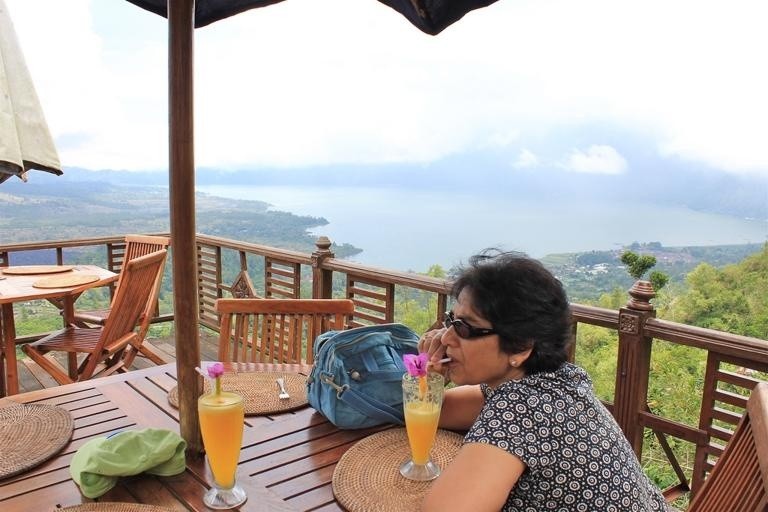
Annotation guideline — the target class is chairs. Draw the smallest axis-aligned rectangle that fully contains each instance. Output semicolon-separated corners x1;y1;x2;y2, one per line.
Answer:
214;297;354;364
21;249;167;387
685;379;768;511
60;234;171;373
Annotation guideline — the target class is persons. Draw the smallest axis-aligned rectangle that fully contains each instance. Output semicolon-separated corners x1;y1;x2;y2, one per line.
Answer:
417;248;673;512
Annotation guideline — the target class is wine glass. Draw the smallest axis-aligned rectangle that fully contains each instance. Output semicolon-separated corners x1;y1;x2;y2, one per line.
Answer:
399;367;443;484
195;393;249;509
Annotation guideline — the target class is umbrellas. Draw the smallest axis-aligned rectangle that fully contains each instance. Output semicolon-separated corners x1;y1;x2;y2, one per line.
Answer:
0;0;63;281
126;0;500;454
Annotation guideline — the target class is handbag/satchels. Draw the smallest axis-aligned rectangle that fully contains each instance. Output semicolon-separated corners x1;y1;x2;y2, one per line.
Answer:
305;320;427;435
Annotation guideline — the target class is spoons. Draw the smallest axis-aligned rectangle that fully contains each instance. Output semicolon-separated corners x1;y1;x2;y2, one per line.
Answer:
273;374;289;402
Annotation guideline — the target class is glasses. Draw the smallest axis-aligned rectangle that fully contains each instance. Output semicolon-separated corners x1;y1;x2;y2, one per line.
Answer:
444;309;500;341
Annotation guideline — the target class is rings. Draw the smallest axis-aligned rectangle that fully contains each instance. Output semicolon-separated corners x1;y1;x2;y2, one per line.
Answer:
423;333;432;341
